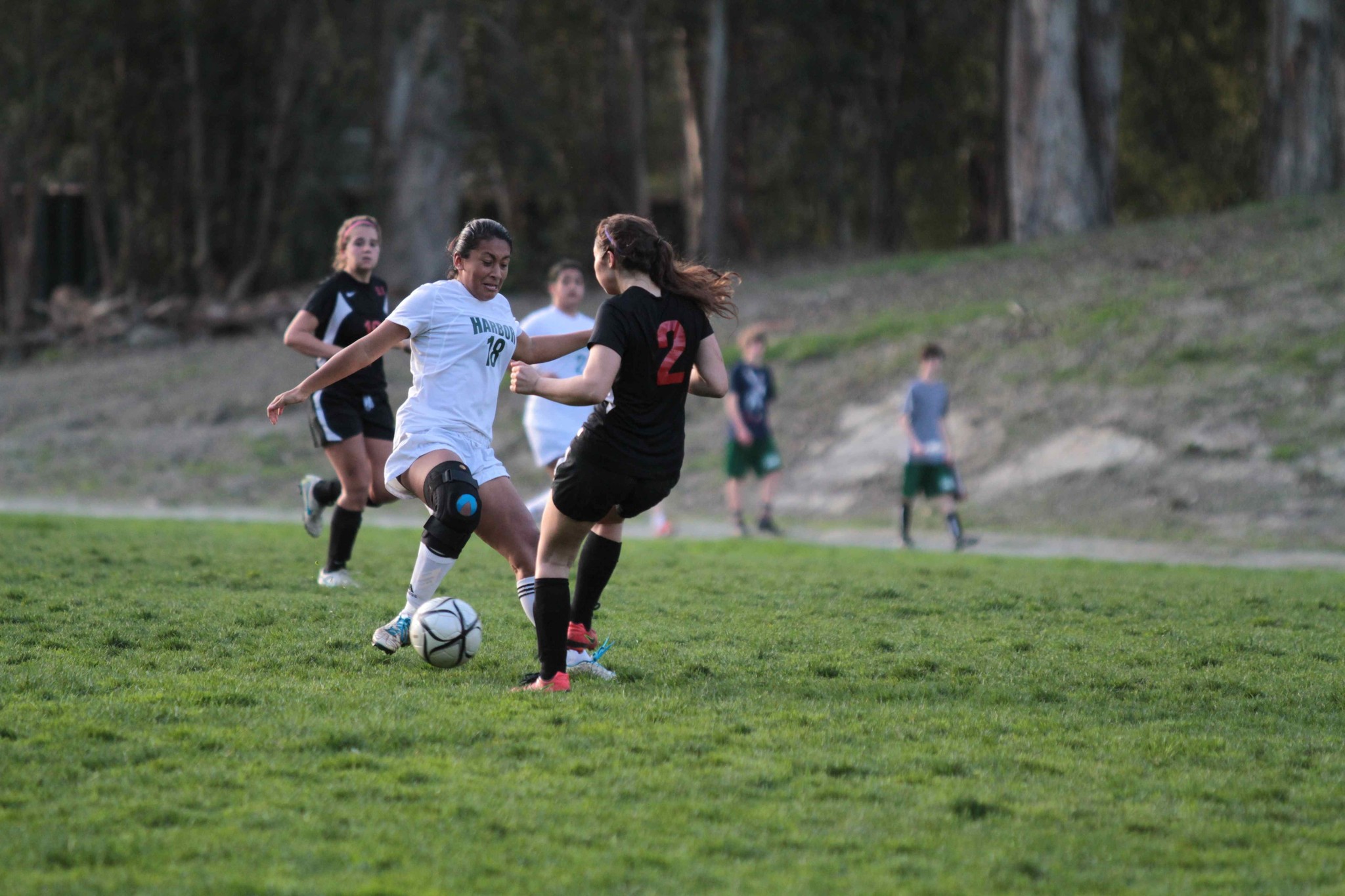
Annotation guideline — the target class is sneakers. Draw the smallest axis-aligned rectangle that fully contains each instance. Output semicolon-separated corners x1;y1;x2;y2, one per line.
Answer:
511;671;571;694
371;614;412;655
566;621;598;650
317;568;347;588
298;474;325;539
566;660;617;682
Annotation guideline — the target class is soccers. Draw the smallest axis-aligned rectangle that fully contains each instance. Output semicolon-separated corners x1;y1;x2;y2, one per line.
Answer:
409;596;483;669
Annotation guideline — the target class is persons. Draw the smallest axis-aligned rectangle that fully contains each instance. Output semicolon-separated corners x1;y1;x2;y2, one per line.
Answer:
284;216;402;586
898;343;977;551
507;211;736;695
264;219;618;682
720;322;786;538
514;259;617;519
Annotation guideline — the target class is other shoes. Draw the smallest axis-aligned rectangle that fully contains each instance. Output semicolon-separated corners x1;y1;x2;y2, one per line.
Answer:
657;519;980;553
